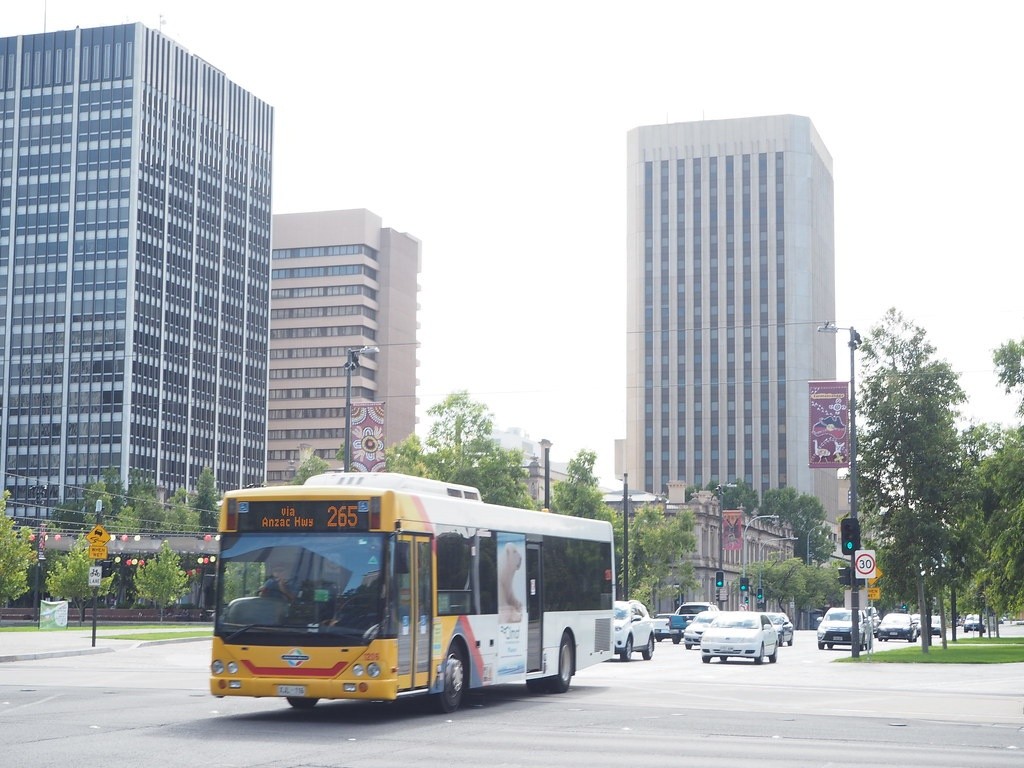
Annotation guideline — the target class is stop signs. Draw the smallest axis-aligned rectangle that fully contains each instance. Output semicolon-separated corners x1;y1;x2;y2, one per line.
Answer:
855;553;875;574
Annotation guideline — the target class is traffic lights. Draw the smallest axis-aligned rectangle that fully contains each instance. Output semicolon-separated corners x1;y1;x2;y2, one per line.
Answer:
745;597;748;604
758;538;798;589
841;518;860;555
838;567;851;585
715;594;719;601
740;578;748;591
716;571;723;587
757;588;762;600
902;605;906;609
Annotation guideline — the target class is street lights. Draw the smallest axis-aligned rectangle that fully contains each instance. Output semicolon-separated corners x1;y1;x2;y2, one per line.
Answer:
344;345;553;509
816;320;861;658
718;483;779;578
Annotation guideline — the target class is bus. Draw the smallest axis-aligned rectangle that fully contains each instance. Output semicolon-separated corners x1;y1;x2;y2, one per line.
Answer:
210;473;614;712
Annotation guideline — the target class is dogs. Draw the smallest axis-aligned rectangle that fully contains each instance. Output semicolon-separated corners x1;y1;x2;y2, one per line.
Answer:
498;542;525;624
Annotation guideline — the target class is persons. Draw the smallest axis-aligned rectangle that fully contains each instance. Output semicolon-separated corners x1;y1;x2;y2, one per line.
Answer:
260;561;300;604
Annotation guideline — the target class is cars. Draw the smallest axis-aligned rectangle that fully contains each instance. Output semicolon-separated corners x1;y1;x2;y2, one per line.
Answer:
963;614;987;633
911;615;921;635
877;613;919;642
930;616;942;638
816;607;881;651
614;601;794;665
